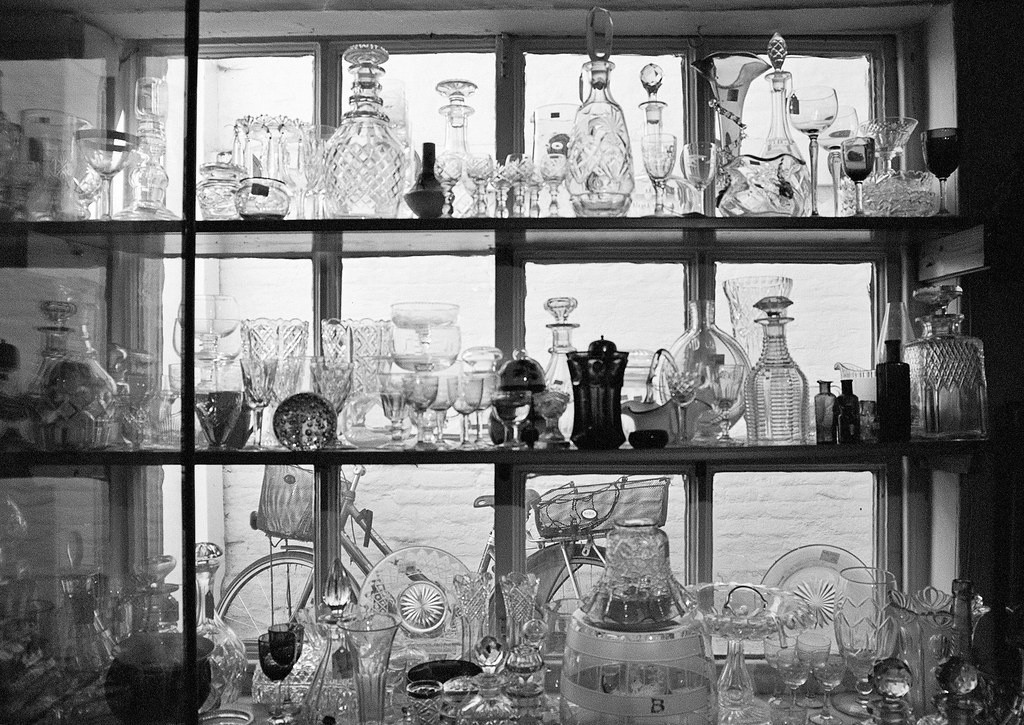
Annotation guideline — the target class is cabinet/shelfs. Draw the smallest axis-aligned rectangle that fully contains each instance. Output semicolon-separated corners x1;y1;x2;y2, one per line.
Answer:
0;1;1024;724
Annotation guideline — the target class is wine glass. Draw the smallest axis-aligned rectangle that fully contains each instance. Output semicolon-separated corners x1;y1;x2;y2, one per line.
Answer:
258;633;295;724
764;638;799;709
809;649;847;724
269;623;304;716
1;87;961;219
770;650;812;718
797;633;831;708
0;279;991;454
828;568;901;716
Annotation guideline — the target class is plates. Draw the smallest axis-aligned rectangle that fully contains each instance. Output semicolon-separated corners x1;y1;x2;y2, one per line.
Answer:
1;542;1024;725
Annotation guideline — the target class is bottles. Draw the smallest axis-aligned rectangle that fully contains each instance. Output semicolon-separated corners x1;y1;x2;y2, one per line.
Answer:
435;80;491;219
759;34;812;217
744;296;809;446
568;7;635;217
660;301;750;443
814;380;836;444
836;379;861;444
558;518;719;725
403;142;446;217
876;340;910;444
931;578;984;725
538;298;581;452
317;45;406;220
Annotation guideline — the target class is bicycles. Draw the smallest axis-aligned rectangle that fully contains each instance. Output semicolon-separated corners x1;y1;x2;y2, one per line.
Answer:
214;464;612;653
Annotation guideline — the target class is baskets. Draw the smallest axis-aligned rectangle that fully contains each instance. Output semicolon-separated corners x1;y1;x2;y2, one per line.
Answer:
530;478;669;538
256;463;353;541
